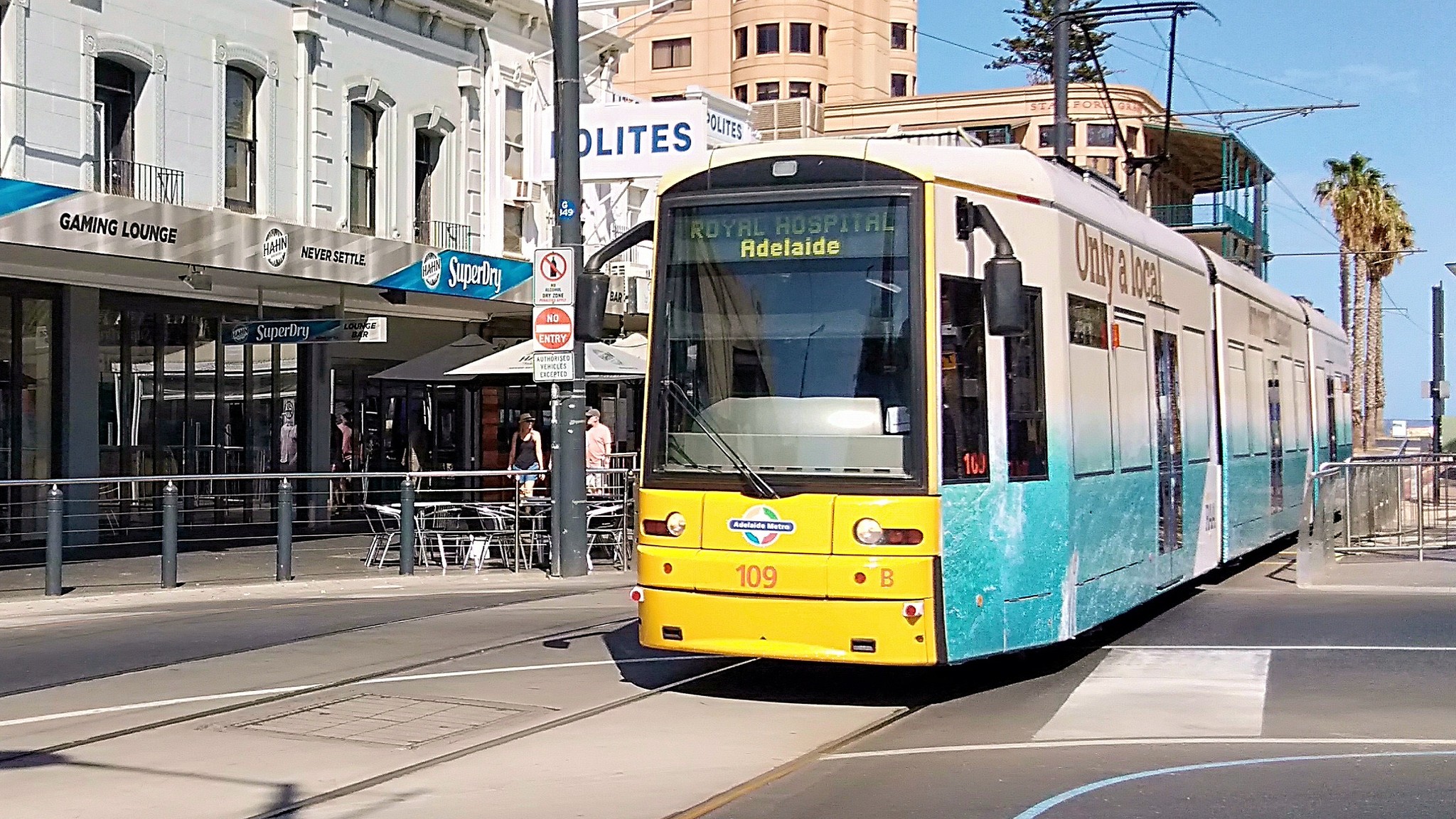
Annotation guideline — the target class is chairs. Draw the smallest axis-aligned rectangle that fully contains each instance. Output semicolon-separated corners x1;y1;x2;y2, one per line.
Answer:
359;500;634;573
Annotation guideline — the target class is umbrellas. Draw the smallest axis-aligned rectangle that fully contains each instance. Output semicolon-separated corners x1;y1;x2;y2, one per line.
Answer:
443;342;646;375
586;334;648;381
368;334;535;502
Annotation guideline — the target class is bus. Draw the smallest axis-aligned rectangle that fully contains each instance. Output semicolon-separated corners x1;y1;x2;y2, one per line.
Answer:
575;1;1354;668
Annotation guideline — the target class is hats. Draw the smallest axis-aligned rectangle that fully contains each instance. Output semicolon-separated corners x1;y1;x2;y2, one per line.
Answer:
585;409;601;418
518;413;536;424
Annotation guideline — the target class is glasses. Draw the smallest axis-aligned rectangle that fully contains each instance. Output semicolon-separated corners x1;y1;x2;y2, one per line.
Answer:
526;420;534;423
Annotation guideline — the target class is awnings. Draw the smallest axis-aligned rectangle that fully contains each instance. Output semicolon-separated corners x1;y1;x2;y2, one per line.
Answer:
143;340;297;377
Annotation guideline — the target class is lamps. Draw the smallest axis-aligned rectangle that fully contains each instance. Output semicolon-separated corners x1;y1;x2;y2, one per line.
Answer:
378;289;406;304
178;265;212;290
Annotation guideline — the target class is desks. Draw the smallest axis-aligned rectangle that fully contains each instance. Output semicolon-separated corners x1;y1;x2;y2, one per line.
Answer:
391;502;451;567
508;504;551;569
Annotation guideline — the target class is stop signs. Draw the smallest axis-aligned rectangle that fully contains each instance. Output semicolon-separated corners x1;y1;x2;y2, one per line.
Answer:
534;308;571;349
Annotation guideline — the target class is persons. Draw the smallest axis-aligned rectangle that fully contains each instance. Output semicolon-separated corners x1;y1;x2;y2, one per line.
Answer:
337;412;352;510
280;425;296;507
3;368;49;535
585;409;612;494
507;413;545;513
548;431;590;475
330;414;343;471
